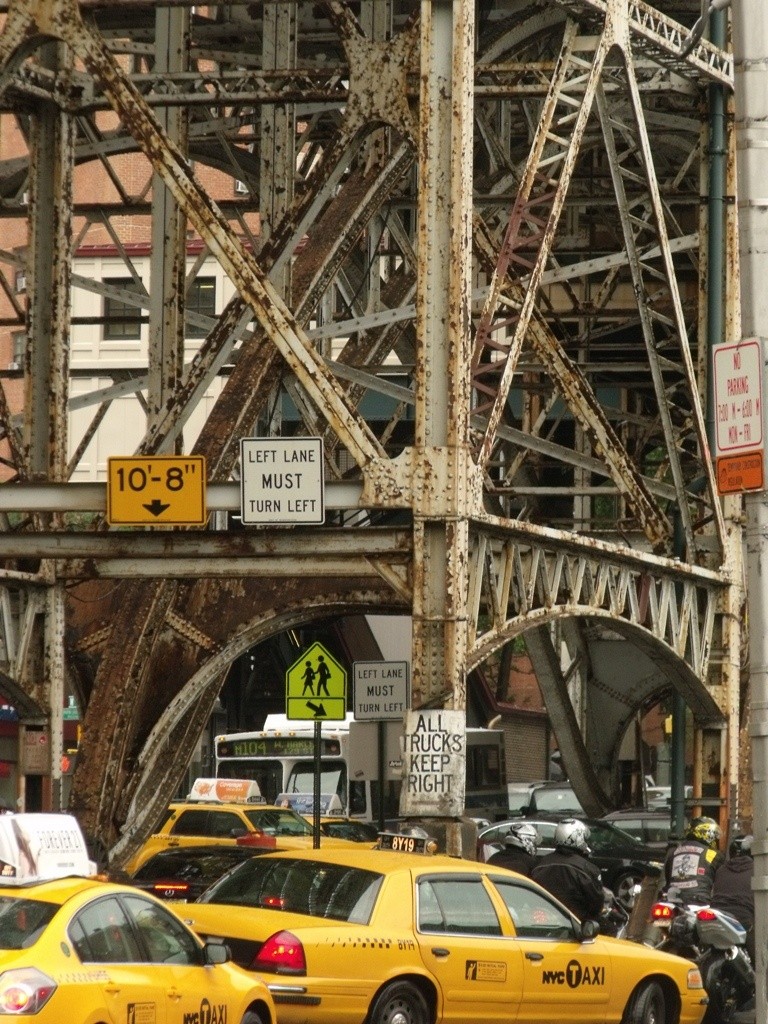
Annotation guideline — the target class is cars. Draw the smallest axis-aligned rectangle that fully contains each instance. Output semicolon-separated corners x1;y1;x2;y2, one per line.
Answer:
116;777;756;1024
154;832;713;1024
1;811;277;1024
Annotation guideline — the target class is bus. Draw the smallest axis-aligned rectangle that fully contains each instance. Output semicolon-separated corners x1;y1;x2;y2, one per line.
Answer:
211;712;509;821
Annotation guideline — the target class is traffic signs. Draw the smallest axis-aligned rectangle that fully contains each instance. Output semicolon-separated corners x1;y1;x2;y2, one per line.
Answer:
107;457;210;526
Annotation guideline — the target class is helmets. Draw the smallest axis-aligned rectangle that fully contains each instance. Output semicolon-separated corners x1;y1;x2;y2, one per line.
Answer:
687;816;721;849
554;817;592;854
503;822;541;858
730;833;754;858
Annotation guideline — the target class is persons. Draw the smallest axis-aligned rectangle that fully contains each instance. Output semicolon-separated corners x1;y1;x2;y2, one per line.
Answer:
530;818;618;938
663;816;755;970
486;824;543;879
